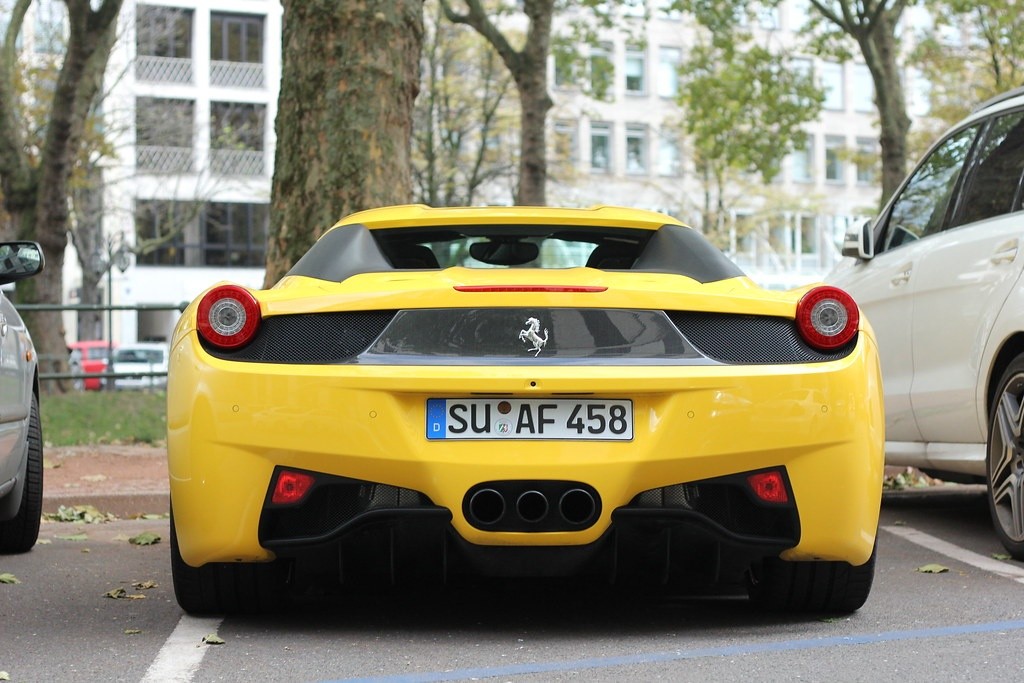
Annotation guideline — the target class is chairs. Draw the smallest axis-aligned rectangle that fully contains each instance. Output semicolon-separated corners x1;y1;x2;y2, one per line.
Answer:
584;242;645;270
386;245;441;270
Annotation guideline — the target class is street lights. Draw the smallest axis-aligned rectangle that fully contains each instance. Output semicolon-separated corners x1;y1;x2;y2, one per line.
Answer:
106;229;130;391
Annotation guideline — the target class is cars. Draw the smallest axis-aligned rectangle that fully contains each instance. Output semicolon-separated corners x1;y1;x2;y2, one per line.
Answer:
0;240;48;552
171;201;888;620
829;86;1024;559
62;341;169;389
